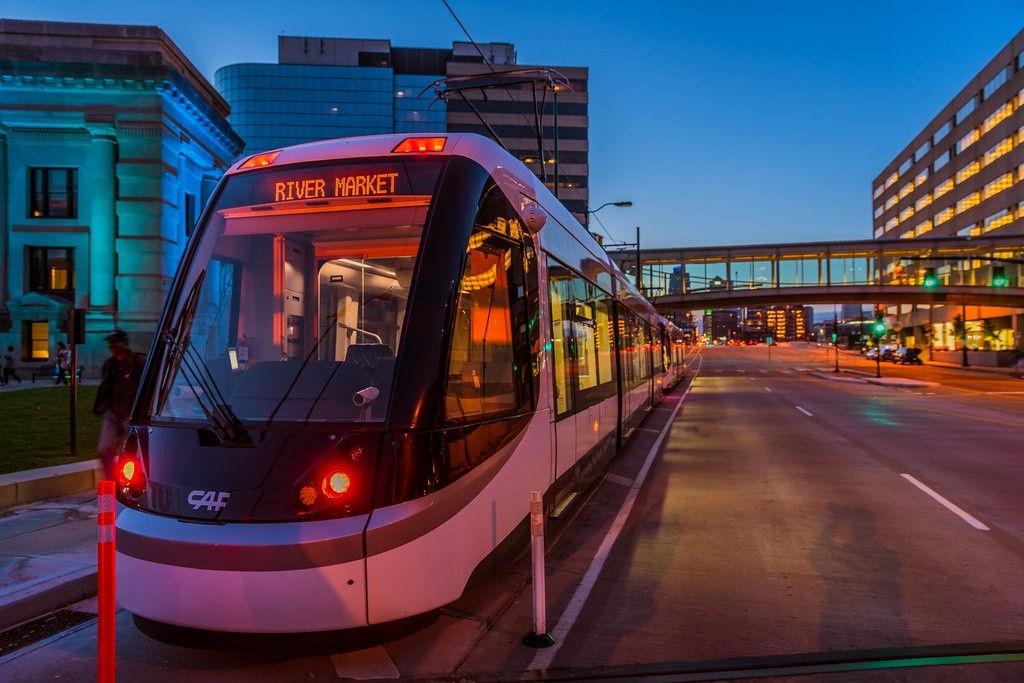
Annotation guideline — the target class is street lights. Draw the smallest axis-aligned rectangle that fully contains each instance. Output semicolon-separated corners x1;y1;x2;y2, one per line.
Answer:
574;202;634;231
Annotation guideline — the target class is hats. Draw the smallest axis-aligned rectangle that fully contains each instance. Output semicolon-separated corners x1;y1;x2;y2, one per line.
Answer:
103;330;127;341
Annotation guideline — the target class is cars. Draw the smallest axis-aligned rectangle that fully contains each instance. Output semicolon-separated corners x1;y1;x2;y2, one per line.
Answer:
893;347;922;365
866;348;895;362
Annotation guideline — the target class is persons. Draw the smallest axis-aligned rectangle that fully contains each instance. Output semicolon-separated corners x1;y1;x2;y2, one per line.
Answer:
0;345;21;387
54;341;70;385
91;330;147;469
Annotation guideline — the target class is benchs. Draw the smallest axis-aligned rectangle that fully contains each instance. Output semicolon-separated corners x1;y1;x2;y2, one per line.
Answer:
31;364;85;385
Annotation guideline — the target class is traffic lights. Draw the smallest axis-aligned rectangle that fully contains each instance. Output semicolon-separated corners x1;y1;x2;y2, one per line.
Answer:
832;333;839;344
876;315;884;334
924;279;935;288
993;279;1004;287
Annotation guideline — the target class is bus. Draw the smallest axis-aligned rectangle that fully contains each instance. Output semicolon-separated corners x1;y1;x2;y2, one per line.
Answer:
113;68;687;633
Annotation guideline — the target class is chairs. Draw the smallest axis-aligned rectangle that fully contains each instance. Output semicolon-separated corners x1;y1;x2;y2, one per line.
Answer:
344;338;394;374
